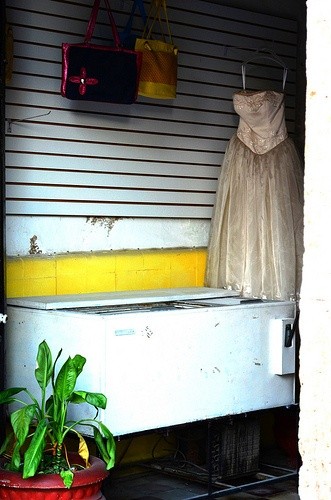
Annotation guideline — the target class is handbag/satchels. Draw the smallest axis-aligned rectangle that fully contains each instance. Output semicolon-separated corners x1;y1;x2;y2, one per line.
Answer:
132;0;177;101
60;0;140;105
118;1;153;52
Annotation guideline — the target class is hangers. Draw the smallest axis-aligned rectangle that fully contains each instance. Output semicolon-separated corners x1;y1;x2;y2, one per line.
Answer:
243;46;293;71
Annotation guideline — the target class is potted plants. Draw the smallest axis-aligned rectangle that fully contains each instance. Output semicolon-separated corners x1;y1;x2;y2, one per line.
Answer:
0;339;116;500
